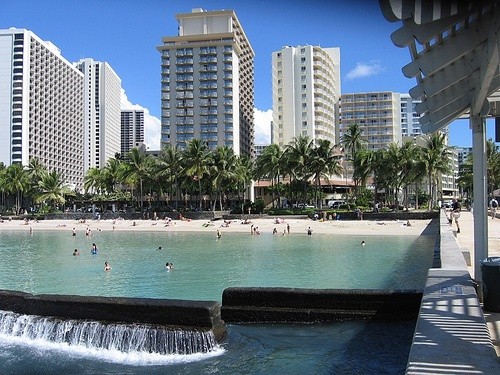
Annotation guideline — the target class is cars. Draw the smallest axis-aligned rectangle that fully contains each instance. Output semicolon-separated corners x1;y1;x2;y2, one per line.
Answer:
442;198;457;208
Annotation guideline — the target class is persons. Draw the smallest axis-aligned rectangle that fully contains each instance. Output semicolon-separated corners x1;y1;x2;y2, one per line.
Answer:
354;207;363;220
90;242;98;254
374;202;380;213
70;212;290;240
157;247;162;250
361;241;366;246
448;197;461;233
305;227;313;236
72;248;80;257
407;220;411;226
0;211;39;237
104;261;112;271
489;197;498;219
165;262;174;270
312;202;342;222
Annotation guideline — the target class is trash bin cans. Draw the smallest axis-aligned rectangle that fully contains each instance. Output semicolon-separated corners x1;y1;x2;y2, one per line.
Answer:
479;256;500;315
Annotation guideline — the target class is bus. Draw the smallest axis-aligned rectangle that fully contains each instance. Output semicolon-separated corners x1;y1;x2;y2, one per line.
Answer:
441;188;459;199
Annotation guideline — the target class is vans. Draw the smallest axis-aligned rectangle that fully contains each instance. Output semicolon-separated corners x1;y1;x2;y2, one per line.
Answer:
327;200;348;210
298;204;308;210
